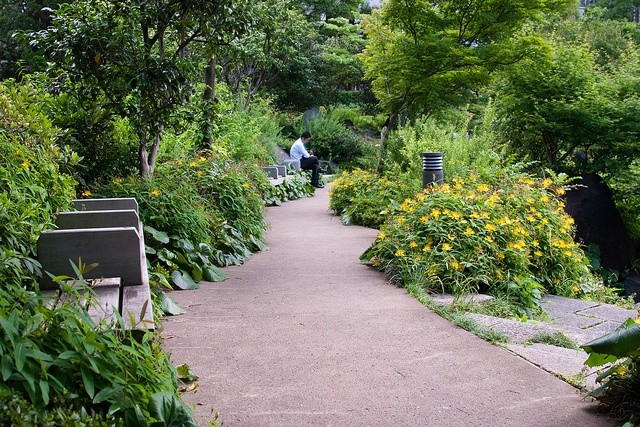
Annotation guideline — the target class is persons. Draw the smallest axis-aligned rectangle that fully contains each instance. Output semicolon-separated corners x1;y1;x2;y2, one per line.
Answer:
290;131;327;188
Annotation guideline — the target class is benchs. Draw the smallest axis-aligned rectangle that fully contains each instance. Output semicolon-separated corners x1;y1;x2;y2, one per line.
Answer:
32;199;155;353
261;165;295;187
283;160;304;171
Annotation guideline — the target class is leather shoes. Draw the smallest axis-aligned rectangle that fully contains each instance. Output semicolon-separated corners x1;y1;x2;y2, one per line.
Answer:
319;167;327;172
313;184;324;188
